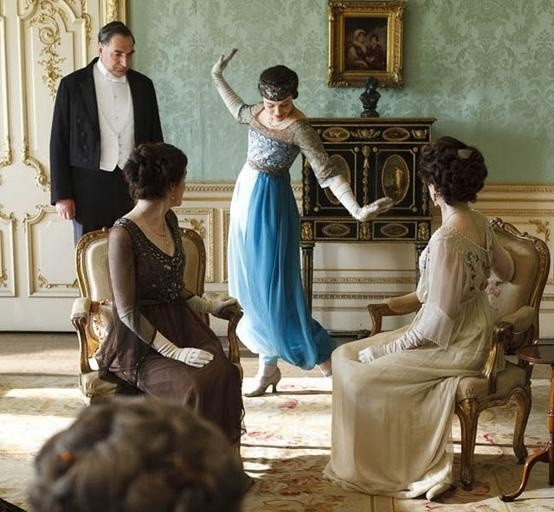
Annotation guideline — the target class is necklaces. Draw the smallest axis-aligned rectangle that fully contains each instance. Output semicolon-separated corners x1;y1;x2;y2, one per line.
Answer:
137;206;168;247
443;210;460;224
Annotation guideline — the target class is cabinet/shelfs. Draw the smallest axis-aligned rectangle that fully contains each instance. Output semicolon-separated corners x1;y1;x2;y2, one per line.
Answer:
291;113;443;322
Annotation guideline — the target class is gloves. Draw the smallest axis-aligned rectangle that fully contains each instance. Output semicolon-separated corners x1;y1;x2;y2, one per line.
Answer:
210;48;246;123
185;284;245;315
356;315;460;365
334;181;397;224
114;307;218;370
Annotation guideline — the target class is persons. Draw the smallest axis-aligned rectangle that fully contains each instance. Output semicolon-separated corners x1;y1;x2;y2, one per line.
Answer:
49;20;164;247
364;34;385;68
322;137;514;501
393;166;404;189
211;49;395;396
349;29;368;69
94;140;254;496
25;395;245;512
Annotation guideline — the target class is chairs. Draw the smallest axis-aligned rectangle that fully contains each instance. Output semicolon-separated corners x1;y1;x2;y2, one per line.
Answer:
359;210;553;492
68;225;248;409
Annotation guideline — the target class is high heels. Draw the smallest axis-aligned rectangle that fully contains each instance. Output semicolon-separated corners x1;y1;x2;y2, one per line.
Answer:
424;466;456;501
243;366;282;400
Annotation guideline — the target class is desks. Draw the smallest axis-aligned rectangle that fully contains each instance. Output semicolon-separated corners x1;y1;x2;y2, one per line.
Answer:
498;341;554;503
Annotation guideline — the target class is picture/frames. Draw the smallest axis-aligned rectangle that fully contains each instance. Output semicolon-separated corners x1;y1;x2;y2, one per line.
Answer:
325;1;406;89
